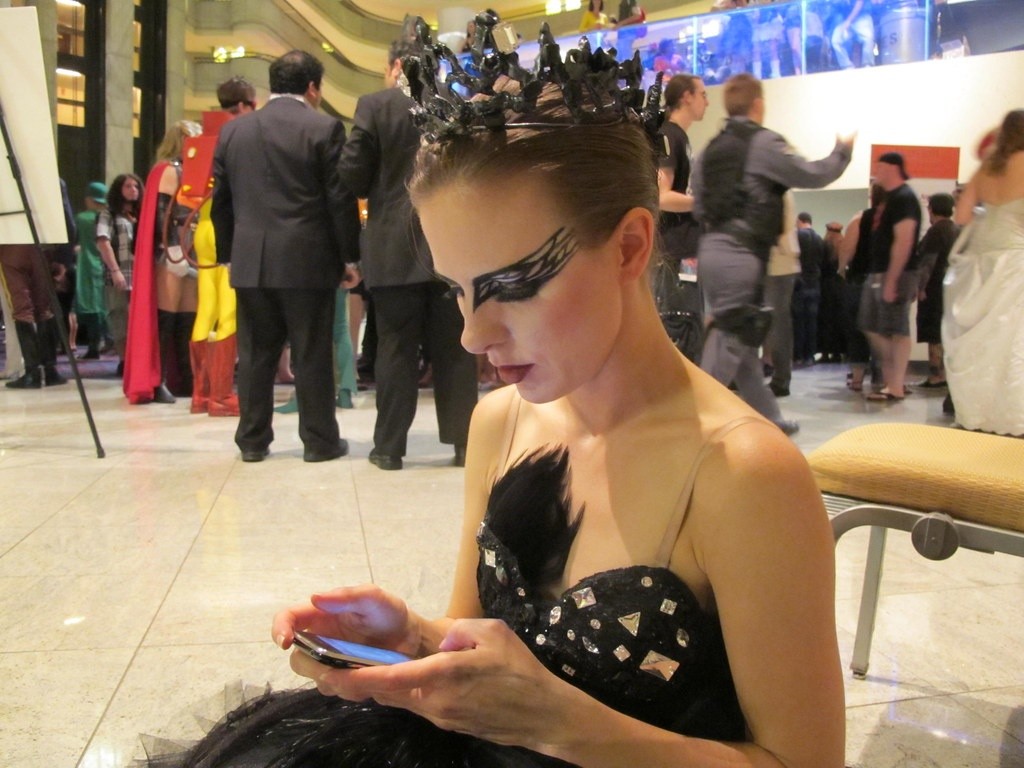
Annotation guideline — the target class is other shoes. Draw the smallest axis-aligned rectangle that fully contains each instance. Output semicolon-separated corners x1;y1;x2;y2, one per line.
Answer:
82;344;99;359
764;350;947;400
781;421;799;435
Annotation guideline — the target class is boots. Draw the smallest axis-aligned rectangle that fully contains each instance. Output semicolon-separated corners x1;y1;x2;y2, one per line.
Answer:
6;319;41;388
205;330;241;416
36;315;67;386
154;308;176;403
188;339;209;413
172;310;194;397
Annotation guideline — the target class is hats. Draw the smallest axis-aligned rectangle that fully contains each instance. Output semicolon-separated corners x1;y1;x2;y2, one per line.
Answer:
826;222;844;233
878;152;908;180
85;182;108;204
930;193;953;214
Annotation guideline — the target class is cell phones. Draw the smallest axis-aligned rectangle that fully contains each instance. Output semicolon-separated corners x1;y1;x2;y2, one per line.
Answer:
294;630;427;669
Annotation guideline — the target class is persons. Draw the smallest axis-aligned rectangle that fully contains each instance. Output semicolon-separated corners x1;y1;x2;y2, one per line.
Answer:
335;39;478;469
71;78;500;418
630;73;1024;438
0;177;78;389
132;71;846;768
211;50;362;464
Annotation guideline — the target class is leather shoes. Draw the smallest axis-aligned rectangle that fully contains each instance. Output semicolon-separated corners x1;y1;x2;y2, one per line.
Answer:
303;439;348;463
243;446;271;462
453;441;466;465
368;447;402;471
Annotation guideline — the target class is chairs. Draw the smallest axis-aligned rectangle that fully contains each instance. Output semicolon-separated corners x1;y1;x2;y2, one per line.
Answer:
806;421;1024;679
